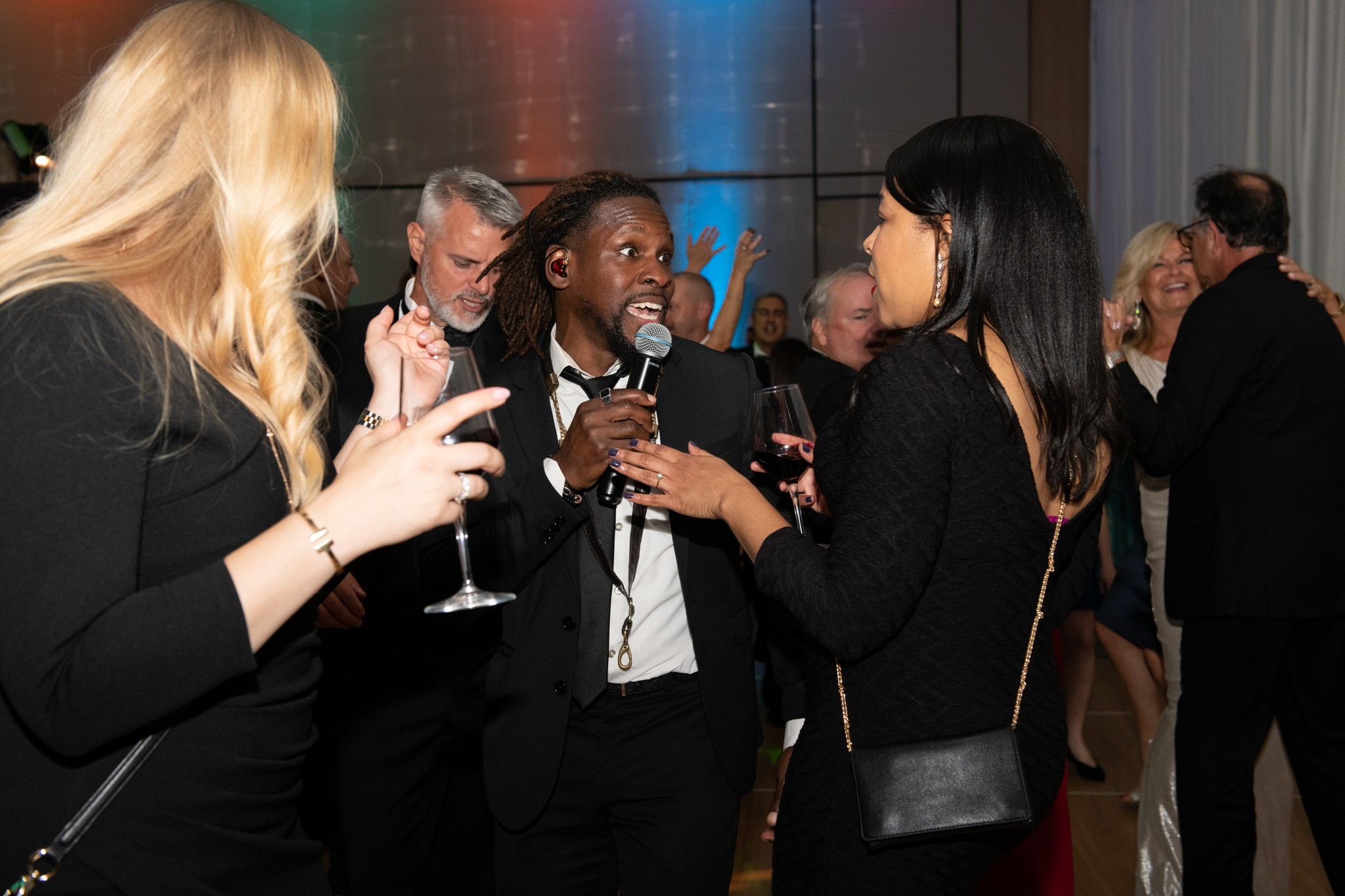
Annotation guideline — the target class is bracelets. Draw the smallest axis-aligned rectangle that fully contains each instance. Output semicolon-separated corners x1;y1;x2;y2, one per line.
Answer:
295;507;345;575
1104;350;1125;370
1327;291;1344;320
357;409;390;429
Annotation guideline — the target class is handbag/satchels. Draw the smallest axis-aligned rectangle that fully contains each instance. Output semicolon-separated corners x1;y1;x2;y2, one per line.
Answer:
850;729;1034;845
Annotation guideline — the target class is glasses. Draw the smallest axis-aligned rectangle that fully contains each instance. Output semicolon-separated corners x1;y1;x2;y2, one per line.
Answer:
1176;217;1225;249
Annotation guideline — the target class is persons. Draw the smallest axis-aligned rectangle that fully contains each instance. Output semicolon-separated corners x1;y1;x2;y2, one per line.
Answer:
0;0;1345;896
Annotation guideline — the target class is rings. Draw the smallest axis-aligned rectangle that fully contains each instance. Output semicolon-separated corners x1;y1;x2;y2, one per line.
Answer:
454;471;471;504
656;472;663;490
599;387;613;405
740;244;747;250
1308;273;1315;286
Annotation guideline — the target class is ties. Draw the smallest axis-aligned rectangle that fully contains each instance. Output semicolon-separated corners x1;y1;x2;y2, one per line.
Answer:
559;366;627;712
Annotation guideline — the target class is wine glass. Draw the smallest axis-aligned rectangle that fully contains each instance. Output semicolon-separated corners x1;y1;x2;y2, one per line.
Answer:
399;346;517;616
754;383;816;537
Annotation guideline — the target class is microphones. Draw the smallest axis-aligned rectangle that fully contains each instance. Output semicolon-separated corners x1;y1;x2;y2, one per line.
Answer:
596;323;674;506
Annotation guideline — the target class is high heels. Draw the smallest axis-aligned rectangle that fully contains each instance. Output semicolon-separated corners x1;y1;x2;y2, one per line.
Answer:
1066;742;1107;781
